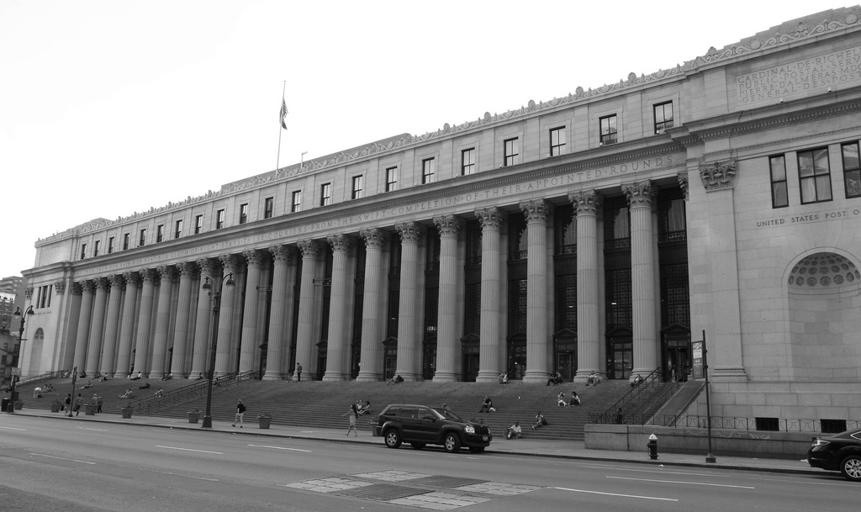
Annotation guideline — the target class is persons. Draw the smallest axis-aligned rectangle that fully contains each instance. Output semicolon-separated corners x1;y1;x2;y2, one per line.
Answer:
498;358;693;389
233;399;245;430
340;404;359;438
506;421;523;440
479;394;494;414
288;364;295;382
533;410;546;430
386;375;395;385
442;402;450;416
569;391;582;405
395;374;404;385
356;399;363;418
358;400;373;416
613;407;625;424
1;363;222;417
297;362;303;382
557;390;569;408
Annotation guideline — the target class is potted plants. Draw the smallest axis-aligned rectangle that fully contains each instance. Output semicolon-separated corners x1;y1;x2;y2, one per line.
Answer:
51;401;60;412
256;412;272;429
121;406;134;418
186;407;201;423
82;403;98;415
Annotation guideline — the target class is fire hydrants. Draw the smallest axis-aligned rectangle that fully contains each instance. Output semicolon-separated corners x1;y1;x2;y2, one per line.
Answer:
647;432;658;460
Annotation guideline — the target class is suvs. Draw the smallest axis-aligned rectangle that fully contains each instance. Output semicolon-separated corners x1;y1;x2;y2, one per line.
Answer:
375;403;492;453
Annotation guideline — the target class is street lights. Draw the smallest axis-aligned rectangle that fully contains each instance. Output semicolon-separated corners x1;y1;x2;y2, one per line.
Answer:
201;272;236;428
10;304;35;400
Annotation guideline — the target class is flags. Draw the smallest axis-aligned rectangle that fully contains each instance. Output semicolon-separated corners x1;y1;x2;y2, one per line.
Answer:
280;93;289;131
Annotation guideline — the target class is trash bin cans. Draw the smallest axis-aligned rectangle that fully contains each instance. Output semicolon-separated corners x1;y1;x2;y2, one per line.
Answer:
2;398;8;411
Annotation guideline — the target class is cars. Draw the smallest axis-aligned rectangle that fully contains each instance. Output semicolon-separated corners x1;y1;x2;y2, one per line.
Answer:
808;428;861;482
